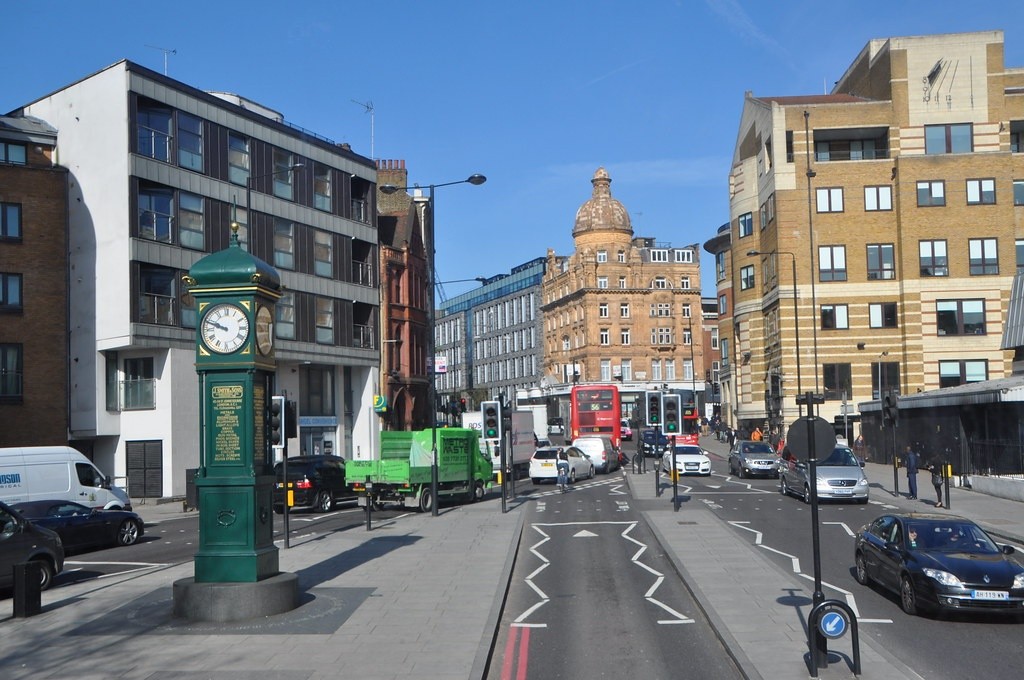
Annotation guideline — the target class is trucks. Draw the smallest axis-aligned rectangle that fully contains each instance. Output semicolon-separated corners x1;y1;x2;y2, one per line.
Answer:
344;428;497;512
461;404;549;476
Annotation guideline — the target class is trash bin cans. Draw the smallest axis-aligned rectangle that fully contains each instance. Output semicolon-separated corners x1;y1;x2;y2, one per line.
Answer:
183;468;205;512
13;561;42;618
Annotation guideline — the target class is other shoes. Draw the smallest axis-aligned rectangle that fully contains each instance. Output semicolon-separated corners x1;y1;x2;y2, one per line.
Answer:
934;502;942;507
907;495;917;500
564;483;569;487
556;483;560;487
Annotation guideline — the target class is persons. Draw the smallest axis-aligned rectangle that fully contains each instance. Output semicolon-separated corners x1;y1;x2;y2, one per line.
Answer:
557;452;569;489
908;527;920;548
696;412;784;458
906;447;918;500
928;453;942;509
946;528;980;549
855;435;868;462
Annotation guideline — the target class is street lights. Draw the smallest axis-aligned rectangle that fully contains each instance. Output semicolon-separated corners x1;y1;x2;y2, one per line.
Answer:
453;324;492;427
825;388;847;439
381;173;489;517
747;249;801;419
796;392;828;670
655;314;697;433
878;351;889;397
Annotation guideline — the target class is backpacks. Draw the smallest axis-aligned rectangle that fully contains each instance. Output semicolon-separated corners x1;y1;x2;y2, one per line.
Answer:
557;451;568;462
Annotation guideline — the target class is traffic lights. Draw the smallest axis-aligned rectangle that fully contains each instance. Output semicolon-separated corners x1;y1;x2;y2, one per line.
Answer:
883;394;897;427
458;398;466;414
646;383;668;426
480;399;501;438
503;407;512;429
662;393;682;435
271;396;285;448
713;382;719;394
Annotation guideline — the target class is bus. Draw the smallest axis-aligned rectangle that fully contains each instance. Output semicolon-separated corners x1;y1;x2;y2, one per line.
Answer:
658;403;698;447
563;386;622;449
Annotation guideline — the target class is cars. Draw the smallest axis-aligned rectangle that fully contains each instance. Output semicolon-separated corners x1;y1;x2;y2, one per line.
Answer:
0;500;145;592
273;454;359;515
855;511;1024;617
528;446;594;483
662;445;711;476
728;440;780;479
549;418;566;435
638;429;670;457
622;418;632;440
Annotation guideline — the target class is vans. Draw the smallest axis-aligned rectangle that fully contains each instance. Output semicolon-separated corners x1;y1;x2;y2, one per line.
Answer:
778;445;870;505
572;437;619;473
0;446;133;510
